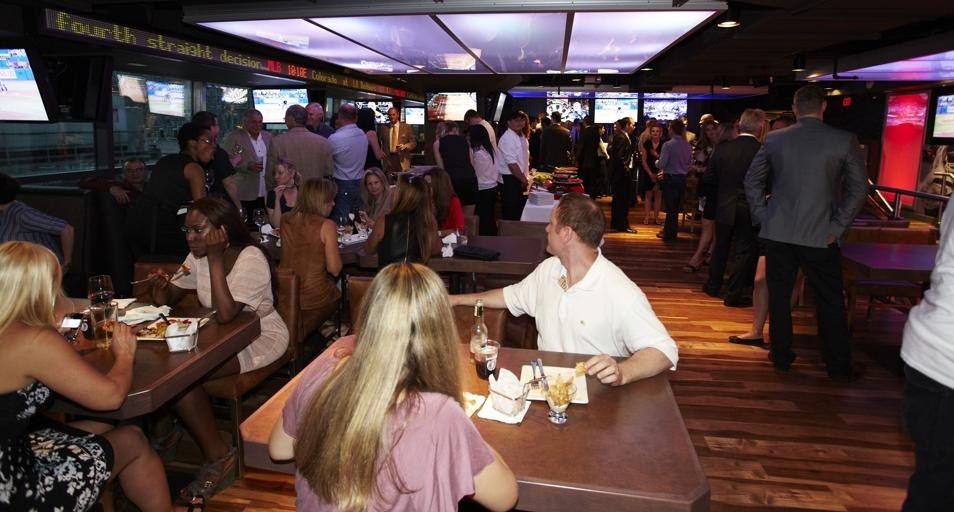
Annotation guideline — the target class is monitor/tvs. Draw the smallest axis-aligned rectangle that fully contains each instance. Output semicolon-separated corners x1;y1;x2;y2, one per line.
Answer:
924;85;954;145
594;92;639;125
642;93;688;124
253;87;311;124
145;79;189;117
355;99;398;127
425;90;478;122
405;106;426;125
0;47;54;124
545;91;591;125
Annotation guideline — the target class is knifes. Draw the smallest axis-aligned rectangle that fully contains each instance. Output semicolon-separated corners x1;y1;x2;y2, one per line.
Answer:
537;357;549;391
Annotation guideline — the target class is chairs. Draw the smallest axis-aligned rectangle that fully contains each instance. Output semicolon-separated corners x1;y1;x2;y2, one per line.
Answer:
465;215;479;237
135;263;300;479
499;221;548;237
455;304;537;347
349;276;374;319
24;186;93;289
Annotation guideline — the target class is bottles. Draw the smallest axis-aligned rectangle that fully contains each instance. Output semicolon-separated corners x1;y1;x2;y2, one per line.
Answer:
469;299;489;364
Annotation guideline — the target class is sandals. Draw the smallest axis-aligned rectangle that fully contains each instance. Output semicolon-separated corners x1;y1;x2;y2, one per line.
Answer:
683;248;714;273
183;494;206;511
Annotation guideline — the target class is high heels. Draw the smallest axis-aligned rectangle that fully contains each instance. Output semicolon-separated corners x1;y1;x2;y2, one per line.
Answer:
177;444;241;506
148;420;183;468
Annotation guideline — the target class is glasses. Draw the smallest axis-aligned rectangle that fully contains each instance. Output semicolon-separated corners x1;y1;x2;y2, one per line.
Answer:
179;225;206;234
194;136;216;145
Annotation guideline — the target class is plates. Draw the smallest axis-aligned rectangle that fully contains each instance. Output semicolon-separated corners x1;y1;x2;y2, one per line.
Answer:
83;296;137;313
136;316;210;342
528;191;554;206
518;363;590;405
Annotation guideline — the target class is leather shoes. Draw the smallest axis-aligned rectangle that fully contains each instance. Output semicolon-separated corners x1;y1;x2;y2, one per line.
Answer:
728;336;765;346
623;227;638;234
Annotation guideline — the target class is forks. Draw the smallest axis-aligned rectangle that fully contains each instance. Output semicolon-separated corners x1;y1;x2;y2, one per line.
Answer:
130;267;183;284
529;360;539;389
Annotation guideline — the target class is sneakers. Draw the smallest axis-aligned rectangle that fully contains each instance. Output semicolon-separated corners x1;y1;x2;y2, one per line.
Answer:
767;352;795;372
657;229;677;243
702;283;721;298
724;296;752;310
827;364;854;383
643;216;661;225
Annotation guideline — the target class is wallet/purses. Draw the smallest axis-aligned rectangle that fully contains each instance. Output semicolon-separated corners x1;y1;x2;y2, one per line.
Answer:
454;243;501;262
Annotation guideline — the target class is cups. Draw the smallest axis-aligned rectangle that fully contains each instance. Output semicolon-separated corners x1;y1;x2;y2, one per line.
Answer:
233;144;242;155
256;155;263;162
474;339;502;380
82;274;122;353
487;384;530;417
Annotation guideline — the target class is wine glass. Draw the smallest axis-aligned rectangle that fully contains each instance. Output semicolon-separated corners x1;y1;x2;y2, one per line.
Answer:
253;208;266;238
337;217;348;249
543;378;577;425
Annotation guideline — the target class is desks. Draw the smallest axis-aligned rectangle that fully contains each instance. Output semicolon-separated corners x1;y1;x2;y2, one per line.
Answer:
520;188;560;224
824;216;938;332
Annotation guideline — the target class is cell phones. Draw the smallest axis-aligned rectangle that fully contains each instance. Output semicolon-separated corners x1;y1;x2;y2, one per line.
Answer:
60;312;85;344
